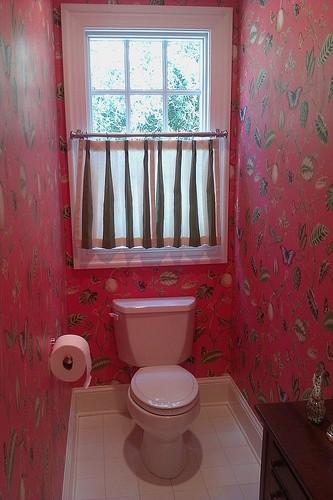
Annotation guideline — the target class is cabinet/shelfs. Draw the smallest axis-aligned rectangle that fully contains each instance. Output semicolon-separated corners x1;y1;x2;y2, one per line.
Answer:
254;399;333;500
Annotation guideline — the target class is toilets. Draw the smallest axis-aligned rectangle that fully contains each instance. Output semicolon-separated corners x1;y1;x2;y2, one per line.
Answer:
106;295;201;480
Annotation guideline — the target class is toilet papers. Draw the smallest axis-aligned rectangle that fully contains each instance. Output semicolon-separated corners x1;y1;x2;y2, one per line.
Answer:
50;333;91;391
